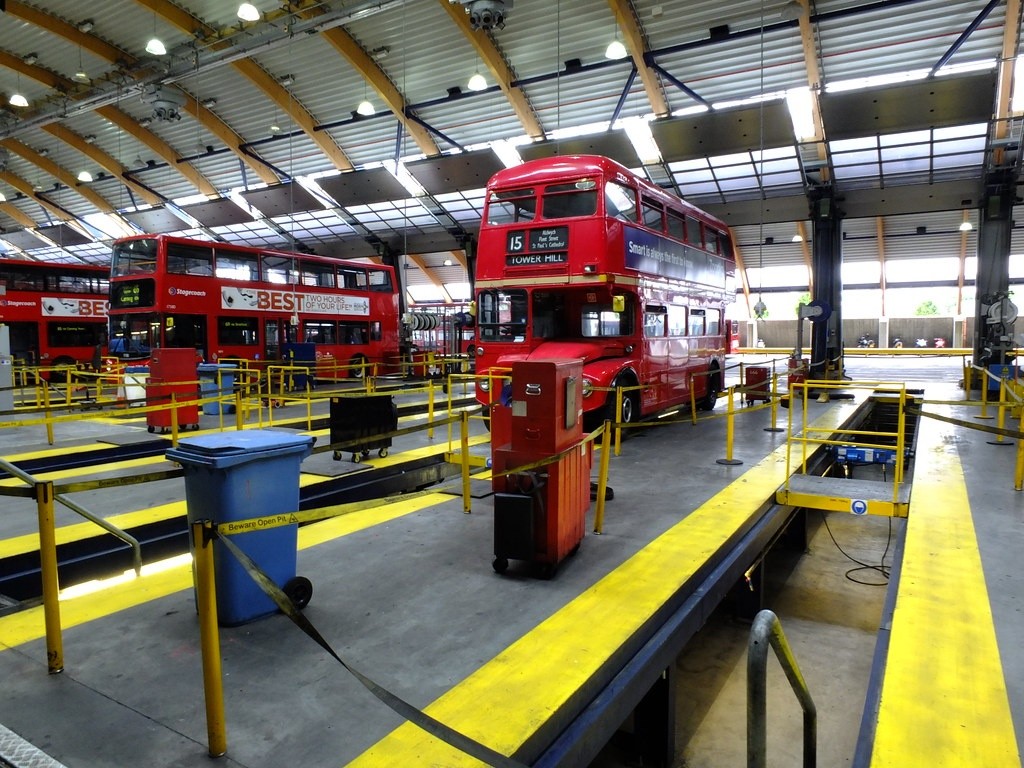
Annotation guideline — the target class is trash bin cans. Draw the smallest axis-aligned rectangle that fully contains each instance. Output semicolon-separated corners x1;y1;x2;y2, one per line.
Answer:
196;362;238;415
164;425;318;630
125;365;149;373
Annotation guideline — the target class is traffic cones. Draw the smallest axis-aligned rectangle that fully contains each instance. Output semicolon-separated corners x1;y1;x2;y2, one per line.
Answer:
109;377;127;409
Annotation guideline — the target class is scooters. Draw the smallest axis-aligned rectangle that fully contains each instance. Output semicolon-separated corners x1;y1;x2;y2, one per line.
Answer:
914;339;929;347
891;336;904;348
856;334;876;348
934;337;946;348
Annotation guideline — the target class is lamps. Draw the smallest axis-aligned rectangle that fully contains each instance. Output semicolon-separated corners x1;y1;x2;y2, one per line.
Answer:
9;72;29;107
32;166;46;193
77;155;93;182
604;12;628;61
145;12;167;54
792;221;803;242
0;193;7;202
959;209;972;231
357;80;376;116
131;103;284;167
468;51;489;92
237;0;261;23
781;0;803;21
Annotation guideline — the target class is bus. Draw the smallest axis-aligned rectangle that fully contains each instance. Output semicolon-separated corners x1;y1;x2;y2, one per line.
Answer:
0;258;157;383
103;231;401;386
467;154;738;444
407;301;512;380
